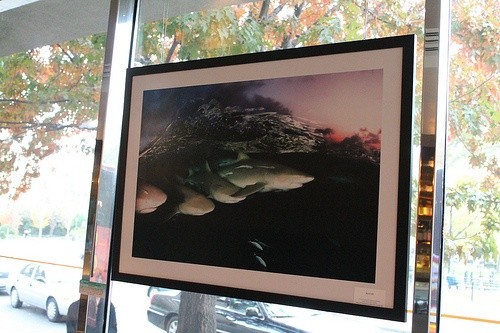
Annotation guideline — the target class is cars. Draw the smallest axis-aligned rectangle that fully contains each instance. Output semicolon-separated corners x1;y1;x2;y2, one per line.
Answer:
147;290;337;333
0;264;12;295
6;262;86;321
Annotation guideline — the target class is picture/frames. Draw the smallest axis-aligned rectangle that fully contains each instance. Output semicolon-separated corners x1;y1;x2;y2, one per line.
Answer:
109;33;418;324
